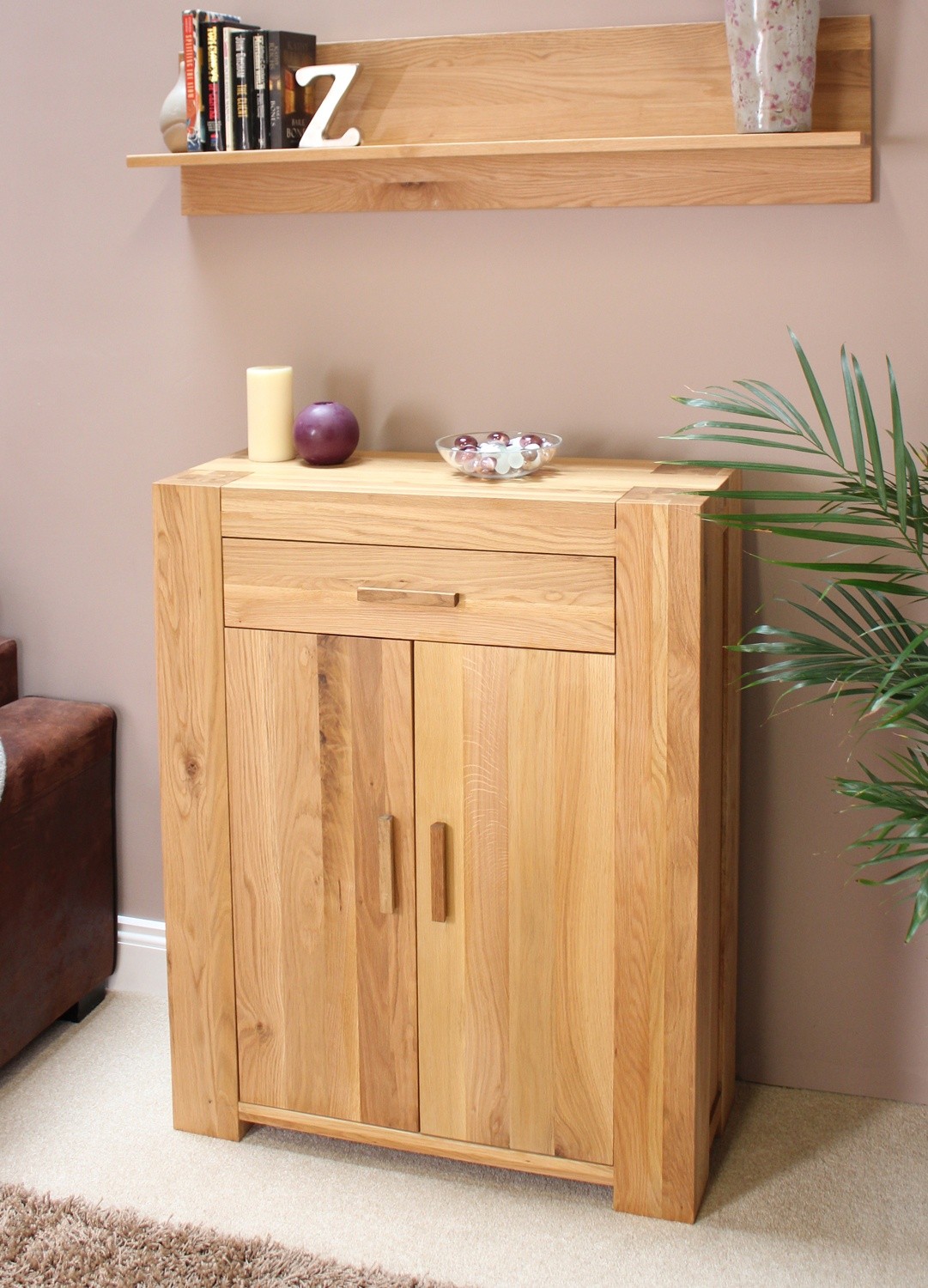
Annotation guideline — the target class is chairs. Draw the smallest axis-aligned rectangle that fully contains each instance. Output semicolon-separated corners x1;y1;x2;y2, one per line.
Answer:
0;636;119;1068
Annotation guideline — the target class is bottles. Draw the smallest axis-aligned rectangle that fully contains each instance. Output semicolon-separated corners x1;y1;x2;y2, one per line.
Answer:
293;401;360;465
159;51;188;153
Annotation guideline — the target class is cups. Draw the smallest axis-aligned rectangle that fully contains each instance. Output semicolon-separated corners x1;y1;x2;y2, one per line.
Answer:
725;1;820;134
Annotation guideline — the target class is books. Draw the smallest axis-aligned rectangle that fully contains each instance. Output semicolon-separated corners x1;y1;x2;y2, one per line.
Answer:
183;9;317;153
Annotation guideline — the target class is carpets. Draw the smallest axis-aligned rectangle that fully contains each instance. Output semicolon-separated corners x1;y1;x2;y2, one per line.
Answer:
0;1181;472;1287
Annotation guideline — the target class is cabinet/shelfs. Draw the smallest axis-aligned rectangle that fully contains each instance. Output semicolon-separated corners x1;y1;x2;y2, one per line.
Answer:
155;447;742;1225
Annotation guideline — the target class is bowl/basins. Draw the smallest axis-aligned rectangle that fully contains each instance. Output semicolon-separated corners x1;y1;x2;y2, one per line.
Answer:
434;430;563;478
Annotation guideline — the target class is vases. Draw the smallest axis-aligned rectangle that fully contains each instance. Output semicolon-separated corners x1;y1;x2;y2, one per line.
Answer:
724;0;820;134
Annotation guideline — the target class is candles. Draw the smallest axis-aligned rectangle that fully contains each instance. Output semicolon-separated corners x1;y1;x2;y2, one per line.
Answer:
246;365;296;463
